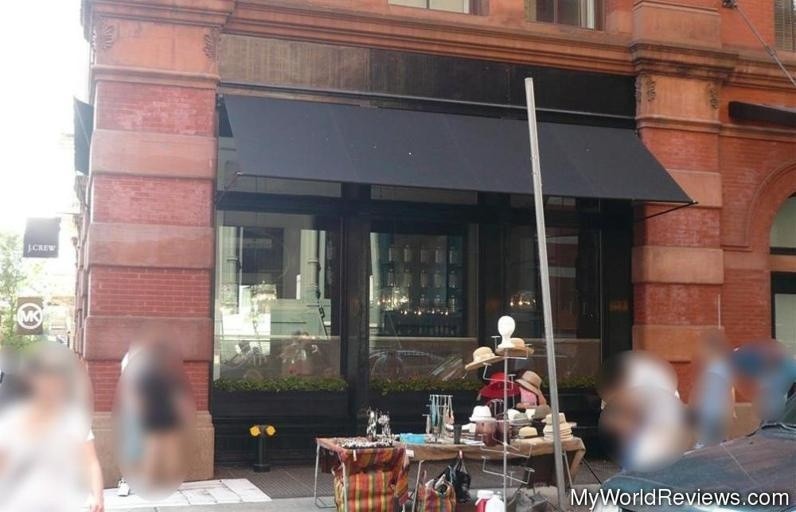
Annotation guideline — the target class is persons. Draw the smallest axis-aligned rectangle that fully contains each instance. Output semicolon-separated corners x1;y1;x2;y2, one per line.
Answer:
497;316;517;349
116;340;186;496
0;359;108;512
596;327;795;466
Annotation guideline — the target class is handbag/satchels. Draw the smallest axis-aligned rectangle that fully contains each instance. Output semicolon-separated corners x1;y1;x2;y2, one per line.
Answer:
330;463;402;512
436;456;471;504
415;468;458;512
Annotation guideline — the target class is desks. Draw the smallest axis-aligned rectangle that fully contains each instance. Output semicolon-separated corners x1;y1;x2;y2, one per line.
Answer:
311;431;586;512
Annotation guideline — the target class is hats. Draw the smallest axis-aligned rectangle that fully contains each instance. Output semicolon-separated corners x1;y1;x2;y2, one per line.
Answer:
510;426;544;443
525;408;536;421
531;404;552;422
513;368;543;397
540;412;578;442
507;408;520;419
468;404;499;422
478;373;520;401
463;345;504;372
509;414;533;426
494;337;534;357
